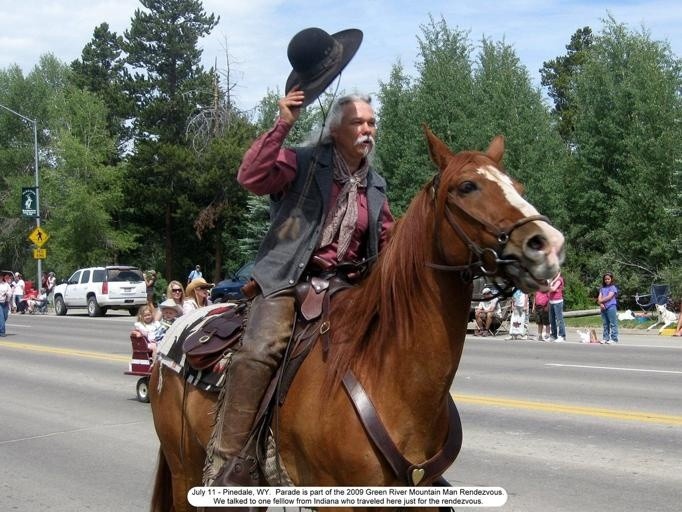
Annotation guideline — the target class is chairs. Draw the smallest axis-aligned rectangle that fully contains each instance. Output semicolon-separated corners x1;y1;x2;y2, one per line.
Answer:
637;282;674;316
477;305;512;337
33;296;51;313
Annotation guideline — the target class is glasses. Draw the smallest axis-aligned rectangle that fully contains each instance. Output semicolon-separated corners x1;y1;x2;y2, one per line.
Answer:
172;288;183;292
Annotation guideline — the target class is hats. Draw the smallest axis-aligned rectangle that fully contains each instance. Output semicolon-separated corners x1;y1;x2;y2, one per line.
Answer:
482;288;491;294
148;270;156;274
158;299;185;315
285;26;363;109
186;278;214;296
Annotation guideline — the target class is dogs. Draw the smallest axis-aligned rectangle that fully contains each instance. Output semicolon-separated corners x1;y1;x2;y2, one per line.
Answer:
647;303;680;335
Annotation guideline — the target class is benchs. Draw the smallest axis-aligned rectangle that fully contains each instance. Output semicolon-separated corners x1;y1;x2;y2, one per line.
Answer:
129;331;153;371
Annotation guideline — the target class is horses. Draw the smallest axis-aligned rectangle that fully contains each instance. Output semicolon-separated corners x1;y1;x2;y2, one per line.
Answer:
148;124;566;512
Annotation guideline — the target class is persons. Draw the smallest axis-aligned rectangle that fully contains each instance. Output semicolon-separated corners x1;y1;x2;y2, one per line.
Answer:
133;265;213;353
597;273;618;342
1;271;56;336
202;83;395;488
475;271;566;342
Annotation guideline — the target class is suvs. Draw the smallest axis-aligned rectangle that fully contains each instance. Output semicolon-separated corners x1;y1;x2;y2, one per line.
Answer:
51;266;148;317
210;259;254;305
468;275;514;333
